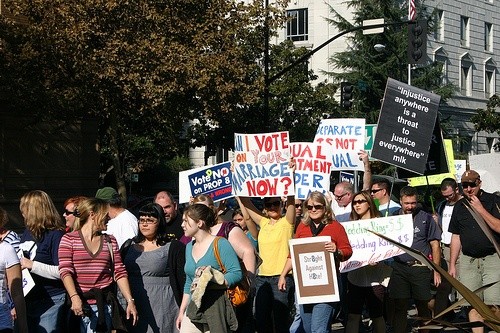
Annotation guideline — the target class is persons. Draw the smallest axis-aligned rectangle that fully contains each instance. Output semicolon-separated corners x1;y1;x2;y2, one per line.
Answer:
58;198;138;333
278;192;353;333
0;190;67;333
63;187;256;333
235;150;464;333
448;170;500;333
176;204;242;333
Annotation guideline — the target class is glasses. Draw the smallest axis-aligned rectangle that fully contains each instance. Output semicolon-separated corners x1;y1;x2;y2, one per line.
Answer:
63;209;74;216
306;205;325;210
266;201;281;208
352;200;368;205
370;189;383;193
337;193;349;200
137;218;158;224
295;204;303;208
463;181;479;188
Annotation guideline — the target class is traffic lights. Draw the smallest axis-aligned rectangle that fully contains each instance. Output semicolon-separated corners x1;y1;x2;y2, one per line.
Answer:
341;82;354;111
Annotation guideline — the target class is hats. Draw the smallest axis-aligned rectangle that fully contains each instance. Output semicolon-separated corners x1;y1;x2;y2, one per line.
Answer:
461;170;480;183
95;187;119;200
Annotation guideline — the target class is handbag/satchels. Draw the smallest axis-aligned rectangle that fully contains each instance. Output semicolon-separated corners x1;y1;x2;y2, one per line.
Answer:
224;221;263;279
214;236;250;308
105;233;118;299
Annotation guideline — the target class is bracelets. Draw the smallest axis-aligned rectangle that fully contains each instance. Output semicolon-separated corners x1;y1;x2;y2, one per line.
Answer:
70;294;78;299
127;299;134;302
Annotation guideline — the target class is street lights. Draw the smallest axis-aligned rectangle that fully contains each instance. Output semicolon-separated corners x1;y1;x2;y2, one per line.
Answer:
374;44;411;86
